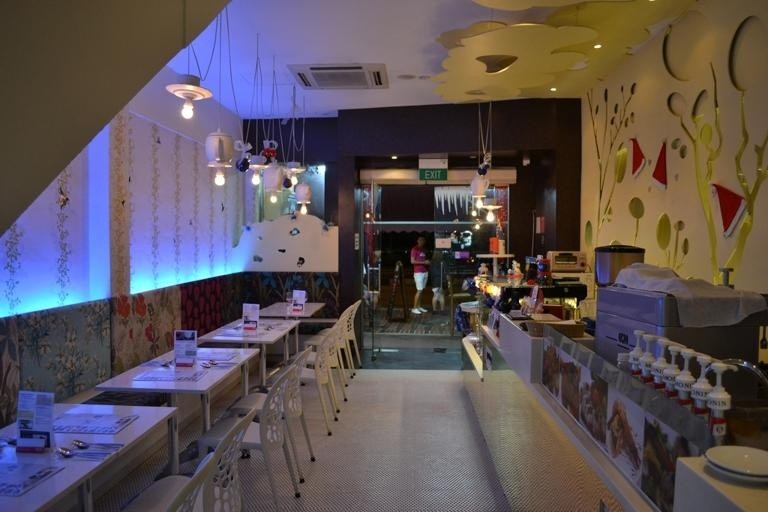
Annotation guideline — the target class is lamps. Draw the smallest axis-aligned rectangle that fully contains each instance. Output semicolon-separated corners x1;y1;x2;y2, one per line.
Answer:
472;102;502;230
166;0;312;216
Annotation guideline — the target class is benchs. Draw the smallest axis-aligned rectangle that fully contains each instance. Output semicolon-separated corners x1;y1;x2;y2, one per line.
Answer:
0;272;341;511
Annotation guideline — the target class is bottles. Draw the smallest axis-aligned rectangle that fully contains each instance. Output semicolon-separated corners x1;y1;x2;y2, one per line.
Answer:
490;237;505;254
617;353;630;373
574;307;581;322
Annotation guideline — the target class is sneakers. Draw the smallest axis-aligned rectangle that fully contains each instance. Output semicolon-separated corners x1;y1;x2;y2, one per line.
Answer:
411;309;422;315
419;307;428;312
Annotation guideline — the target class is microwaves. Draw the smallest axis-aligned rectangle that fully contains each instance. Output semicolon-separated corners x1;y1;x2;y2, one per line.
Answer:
547;250;588;272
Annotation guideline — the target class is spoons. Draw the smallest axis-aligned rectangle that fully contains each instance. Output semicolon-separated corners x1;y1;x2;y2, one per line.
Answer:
201;360;240;368
73;440;125;449
59;447;113;459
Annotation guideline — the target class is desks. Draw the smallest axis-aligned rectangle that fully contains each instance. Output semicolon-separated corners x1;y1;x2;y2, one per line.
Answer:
196;316;302;406
460;300;480;314
94;344;263;460
1;398;182;508
477;252;513;281
255;300;326;362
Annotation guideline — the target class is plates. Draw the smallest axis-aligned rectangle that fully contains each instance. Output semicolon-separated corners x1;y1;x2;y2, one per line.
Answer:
703;444;768;486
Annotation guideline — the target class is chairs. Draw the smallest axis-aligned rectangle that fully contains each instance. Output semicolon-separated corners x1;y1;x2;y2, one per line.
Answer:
445;274;472;337
272;299;361;437
229;345;317;483
116;406;261;511
193;363;305;508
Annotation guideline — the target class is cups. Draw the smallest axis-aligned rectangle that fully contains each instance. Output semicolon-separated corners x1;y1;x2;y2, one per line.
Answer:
286;292;293;304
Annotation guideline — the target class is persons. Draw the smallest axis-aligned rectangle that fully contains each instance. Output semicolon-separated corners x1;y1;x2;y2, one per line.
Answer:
411;235;430;314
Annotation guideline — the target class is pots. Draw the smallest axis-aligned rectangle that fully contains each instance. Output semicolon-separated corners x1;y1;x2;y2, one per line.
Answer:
594;243;645;287
